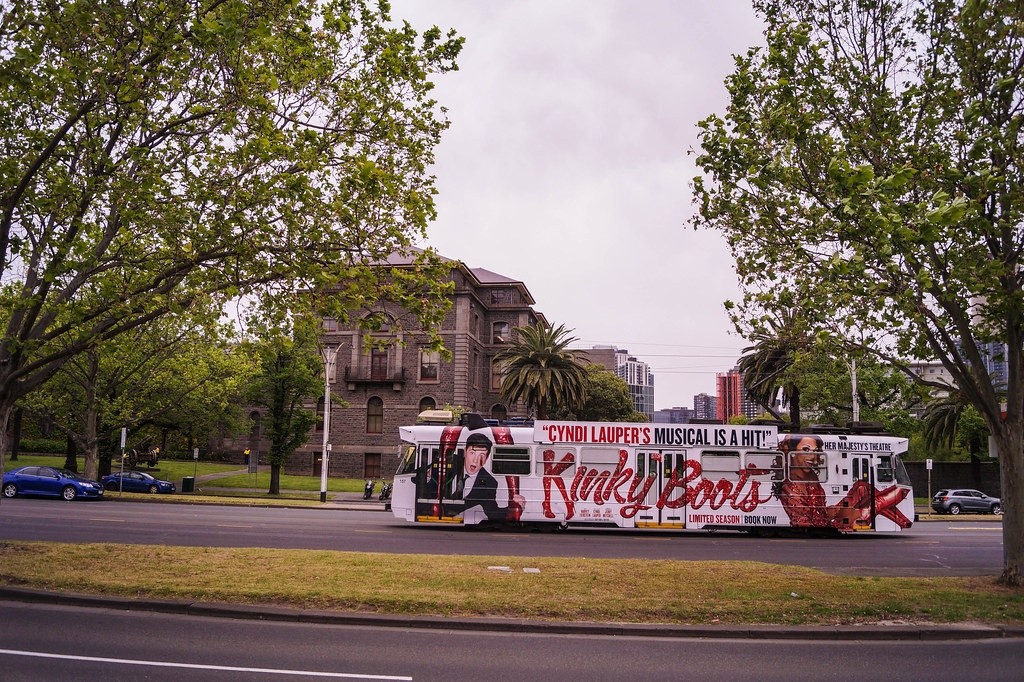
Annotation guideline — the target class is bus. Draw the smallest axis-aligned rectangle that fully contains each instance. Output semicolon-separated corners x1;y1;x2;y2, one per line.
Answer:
386;418;917;536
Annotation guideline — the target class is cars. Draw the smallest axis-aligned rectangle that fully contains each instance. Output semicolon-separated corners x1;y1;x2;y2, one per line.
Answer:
2;464;104;501
103;469;177;494
932;490;1002;515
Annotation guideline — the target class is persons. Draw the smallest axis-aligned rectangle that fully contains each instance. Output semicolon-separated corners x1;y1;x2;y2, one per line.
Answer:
770;436;912;531
413;434;526;521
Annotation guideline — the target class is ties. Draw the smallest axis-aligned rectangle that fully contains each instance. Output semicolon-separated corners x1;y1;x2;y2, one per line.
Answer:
463;474;470;488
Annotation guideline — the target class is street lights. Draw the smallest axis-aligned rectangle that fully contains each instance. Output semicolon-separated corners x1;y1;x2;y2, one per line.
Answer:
292;312;356;502
829;352;859;423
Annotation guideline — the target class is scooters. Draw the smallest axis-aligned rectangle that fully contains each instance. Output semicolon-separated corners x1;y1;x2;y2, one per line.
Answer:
378;481;393;500
362;479;378;500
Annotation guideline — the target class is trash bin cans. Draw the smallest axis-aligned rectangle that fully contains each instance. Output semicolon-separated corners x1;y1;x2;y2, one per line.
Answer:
182;477;195;492
